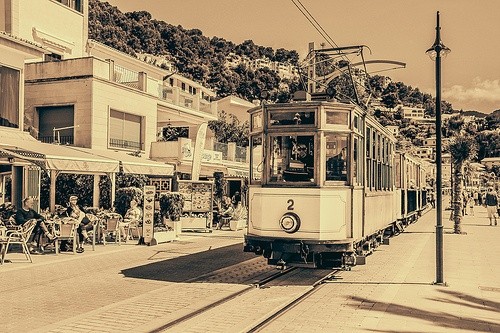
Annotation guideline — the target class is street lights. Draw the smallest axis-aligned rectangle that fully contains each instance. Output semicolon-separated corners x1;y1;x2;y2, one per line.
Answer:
424;10;452;287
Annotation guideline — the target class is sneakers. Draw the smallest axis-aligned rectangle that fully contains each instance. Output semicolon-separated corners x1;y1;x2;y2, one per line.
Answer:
46;233;58;243
84;238;93;243
76;247;85;252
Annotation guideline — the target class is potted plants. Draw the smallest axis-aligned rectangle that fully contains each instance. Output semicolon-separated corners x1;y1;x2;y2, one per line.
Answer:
229;203;247;231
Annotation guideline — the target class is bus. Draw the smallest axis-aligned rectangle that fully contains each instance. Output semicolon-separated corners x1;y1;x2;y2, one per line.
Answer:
242;83;428;271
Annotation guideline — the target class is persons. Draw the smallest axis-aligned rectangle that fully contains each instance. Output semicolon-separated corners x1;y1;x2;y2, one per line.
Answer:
485;187;498;226
214;189;240;230
461;186;500;219
64;195;95;252
15;197;58;255
338;148;355;177
426;189;436;208
121;200;142;241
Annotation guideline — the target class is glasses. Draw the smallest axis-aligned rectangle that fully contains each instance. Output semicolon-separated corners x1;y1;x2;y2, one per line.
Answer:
297;148;307;151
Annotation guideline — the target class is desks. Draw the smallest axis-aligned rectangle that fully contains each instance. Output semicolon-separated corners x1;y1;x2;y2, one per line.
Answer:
45;220;57;253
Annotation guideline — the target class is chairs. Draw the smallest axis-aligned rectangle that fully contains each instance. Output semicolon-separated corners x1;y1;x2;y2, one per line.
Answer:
81;213;99;251
8;214;26;253
5;218;38;265
123;206;143;243
55;216;80;255
100;212;123;246
